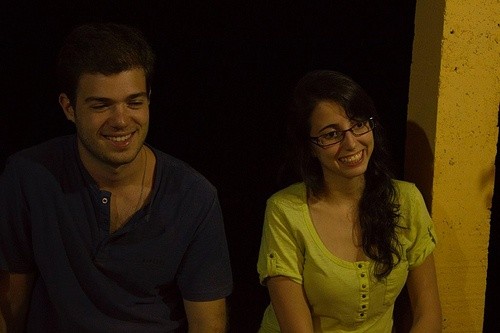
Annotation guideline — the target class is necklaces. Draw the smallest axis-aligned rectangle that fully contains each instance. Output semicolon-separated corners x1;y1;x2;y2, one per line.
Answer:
135;147;147;210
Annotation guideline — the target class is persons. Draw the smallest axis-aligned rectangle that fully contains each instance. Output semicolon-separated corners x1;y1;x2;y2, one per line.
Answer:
1;20;234;333
254;68;444;333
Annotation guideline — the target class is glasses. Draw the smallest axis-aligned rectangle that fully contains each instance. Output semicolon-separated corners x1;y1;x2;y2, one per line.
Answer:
310;116;376;148
